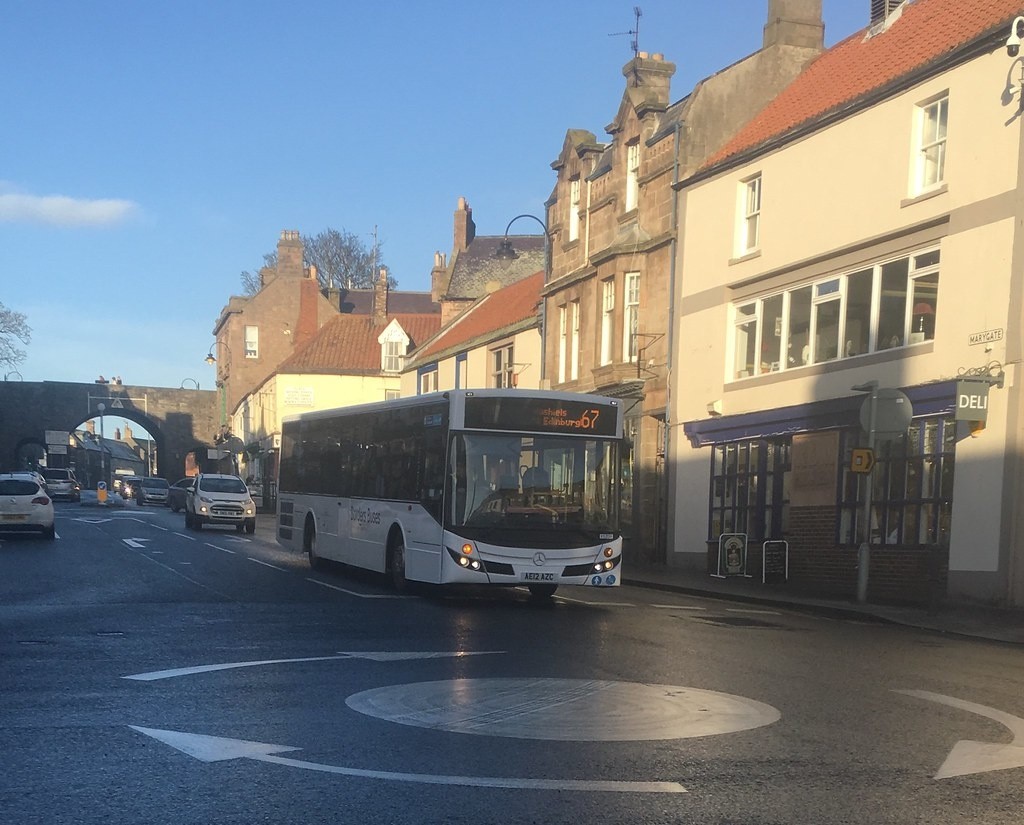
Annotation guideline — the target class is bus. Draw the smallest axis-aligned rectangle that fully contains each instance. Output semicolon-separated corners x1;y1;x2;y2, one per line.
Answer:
275;388;635;598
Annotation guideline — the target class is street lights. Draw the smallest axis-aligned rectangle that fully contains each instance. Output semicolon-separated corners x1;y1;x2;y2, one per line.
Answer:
97;403;105;481
851;380;879;607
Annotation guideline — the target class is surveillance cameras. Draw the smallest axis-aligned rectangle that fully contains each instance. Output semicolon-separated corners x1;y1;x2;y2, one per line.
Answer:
1006;35;1021;57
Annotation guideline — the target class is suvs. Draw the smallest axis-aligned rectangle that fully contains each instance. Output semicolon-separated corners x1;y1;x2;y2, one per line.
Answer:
185;473;257;533
41;467;82;503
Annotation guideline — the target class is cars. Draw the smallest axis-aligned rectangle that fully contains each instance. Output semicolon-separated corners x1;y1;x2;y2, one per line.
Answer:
137;477;171;507
120;476;144;500
168;477;196;512
0;471;50;494
0;474;55;538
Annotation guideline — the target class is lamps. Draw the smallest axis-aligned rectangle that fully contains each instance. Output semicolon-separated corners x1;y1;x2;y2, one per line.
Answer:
179;378;199;391
204;341;231;365
492;213;553;270
